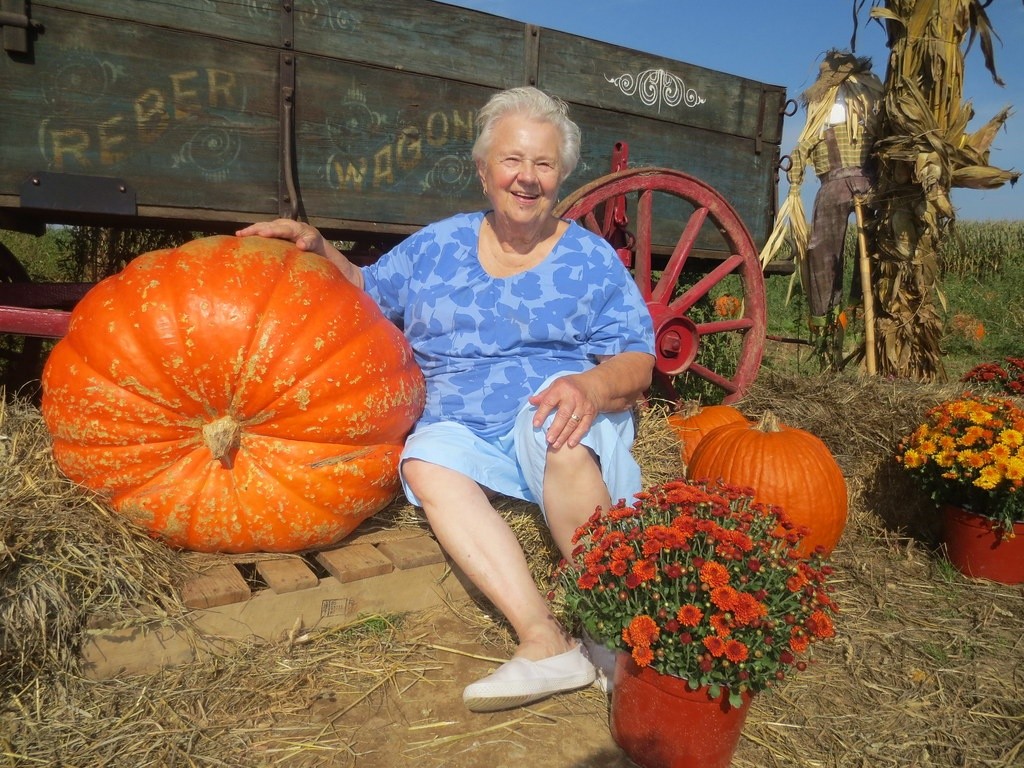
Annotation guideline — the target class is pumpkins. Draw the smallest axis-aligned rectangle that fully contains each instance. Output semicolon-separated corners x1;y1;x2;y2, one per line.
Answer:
714;296;740;317
42;234;427;555
663;398;747;468
807;311;847;335
686;409;847;562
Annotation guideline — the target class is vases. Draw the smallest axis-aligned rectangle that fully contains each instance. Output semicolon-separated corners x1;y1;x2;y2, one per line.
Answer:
609;648;756;768
941;499;1023;585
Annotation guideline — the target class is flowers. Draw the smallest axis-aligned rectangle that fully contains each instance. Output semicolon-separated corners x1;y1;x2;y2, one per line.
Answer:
545;477;839;707
894;390;1024;542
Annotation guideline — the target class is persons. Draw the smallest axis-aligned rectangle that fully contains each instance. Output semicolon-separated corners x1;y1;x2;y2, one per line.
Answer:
234;85;657;713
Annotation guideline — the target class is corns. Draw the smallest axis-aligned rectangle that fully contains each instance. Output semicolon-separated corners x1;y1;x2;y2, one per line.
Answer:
943;101;1020;188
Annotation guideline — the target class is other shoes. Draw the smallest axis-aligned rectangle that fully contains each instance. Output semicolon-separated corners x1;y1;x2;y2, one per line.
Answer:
580;624;616;696
463;638;595;712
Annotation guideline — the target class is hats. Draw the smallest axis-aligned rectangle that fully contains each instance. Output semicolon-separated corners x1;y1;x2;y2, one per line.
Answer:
802;51;874;100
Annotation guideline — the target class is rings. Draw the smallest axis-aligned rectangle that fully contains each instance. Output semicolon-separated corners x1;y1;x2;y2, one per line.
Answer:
571;413;581;422
